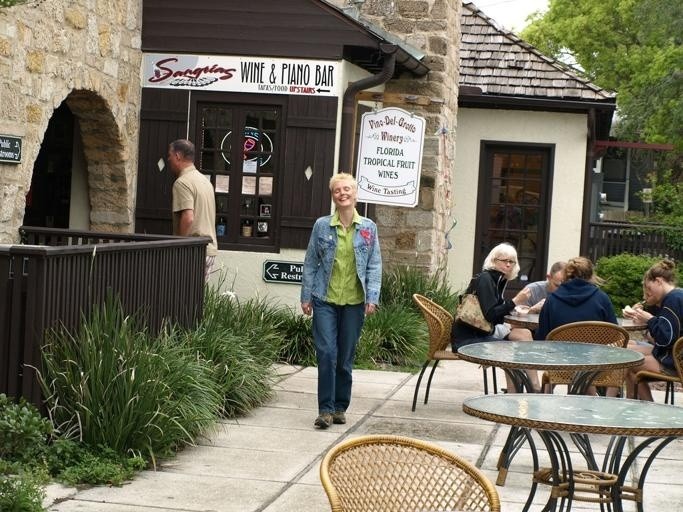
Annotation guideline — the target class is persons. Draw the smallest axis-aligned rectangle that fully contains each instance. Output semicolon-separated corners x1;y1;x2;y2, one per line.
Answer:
535;256;618;397
615;280;661;346
168;138;217;287
480;189;540;281
512;261;567;314
300;172;382;428
449;242;541;392
625;259;682;401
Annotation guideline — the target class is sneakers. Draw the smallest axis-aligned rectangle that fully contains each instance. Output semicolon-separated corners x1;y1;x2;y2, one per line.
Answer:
333;410;346;424
314;411;334;428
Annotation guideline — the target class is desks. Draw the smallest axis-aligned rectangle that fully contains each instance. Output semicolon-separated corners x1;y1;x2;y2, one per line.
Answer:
463;393;682;512
503;314;648;396
458;339;645;486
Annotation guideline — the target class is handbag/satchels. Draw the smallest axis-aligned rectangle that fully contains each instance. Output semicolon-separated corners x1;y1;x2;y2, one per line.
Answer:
454;293;494;335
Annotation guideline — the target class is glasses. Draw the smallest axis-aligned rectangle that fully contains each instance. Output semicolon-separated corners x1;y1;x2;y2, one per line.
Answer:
496;258;517;266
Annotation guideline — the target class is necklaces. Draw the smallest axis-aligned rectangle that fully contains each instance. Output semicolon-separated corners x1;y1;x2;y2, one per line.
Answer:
338;216;352;233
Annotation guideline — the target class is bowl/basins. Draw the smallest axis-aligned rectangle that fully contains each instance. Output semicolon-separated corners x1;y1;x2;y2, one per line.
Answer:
515;305;529;316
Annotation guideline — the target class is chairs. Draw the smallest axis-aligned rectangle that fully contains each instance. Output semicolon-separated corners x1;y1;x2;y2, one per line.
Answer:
540;321;631;396
411;293;497;412
633;337;683;404
320;433;501;512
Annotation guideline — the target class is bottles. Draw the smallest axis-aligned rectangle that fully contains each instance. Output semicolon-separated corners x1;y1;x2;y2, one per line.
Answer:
215;203;226;236
239;204;253;238
